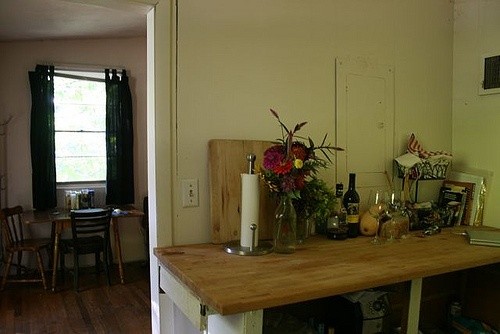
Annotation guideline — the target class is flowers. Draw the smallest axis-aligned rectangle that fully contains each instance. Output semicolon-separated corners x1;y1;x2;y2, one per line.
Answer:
251;108;343;242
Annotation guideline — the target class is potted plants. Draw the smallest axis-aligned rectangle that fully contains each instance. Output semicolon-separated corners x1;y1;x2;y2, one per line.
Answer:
299;177;339;239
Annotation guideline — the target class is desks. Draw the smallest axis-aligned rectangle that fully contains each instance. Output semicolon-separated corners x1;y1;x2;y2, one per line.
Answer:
154;224;500;334
21;205;145;292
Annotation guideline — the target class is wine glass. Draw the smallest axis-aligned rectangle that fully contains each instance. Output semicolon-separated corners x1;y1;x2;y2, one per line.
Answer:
385;192;404;242
368;190;385;243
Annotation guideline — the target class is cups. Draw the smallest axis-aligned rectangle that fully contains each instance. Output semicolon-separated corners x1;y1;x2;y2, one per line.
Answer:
89;190;94;209
71;194;78;210
76;192;81;209
64;191;72;208
81;189;88;208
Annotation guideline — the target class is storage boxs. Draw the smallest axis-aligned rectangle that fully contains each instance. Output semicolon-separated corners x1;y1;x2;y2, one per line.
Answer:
398;177;446;204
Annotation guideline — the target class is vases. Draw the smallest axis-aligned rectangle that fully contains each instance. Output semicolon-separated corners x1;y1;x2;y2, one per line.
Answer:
274;191;298;253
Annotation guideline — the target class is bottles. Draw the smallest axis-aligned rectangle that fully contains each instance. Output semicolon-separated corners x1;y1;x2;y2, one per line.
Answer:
327;184;346;240
401;207;409;234
342;174;360;239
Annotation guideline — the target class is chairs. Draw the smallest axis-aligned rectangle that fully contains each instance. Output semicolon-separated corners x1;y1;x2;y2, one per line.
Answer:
58;207;113;293
0;205;50;291
